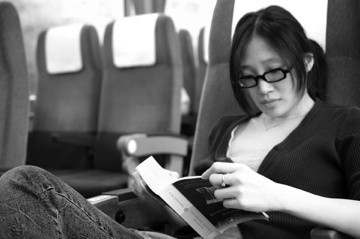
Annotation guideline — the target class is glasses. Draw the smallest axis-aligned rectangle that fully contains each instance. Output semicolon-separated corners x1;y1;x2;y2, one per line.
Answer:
236;60;295;89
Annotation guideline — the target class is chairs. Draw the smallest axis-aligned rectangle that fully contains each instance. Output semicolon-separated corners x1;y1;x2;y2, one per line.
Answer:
0;0;360;239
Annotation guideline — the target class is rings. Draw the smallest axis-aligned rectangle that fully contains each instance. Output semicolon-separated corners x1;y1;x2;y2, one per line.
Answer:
221;174;226;187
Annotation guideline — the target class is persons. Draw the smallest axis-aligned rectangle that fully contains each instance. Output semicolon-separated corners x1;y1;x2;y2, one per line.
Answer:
0;7;360;239
